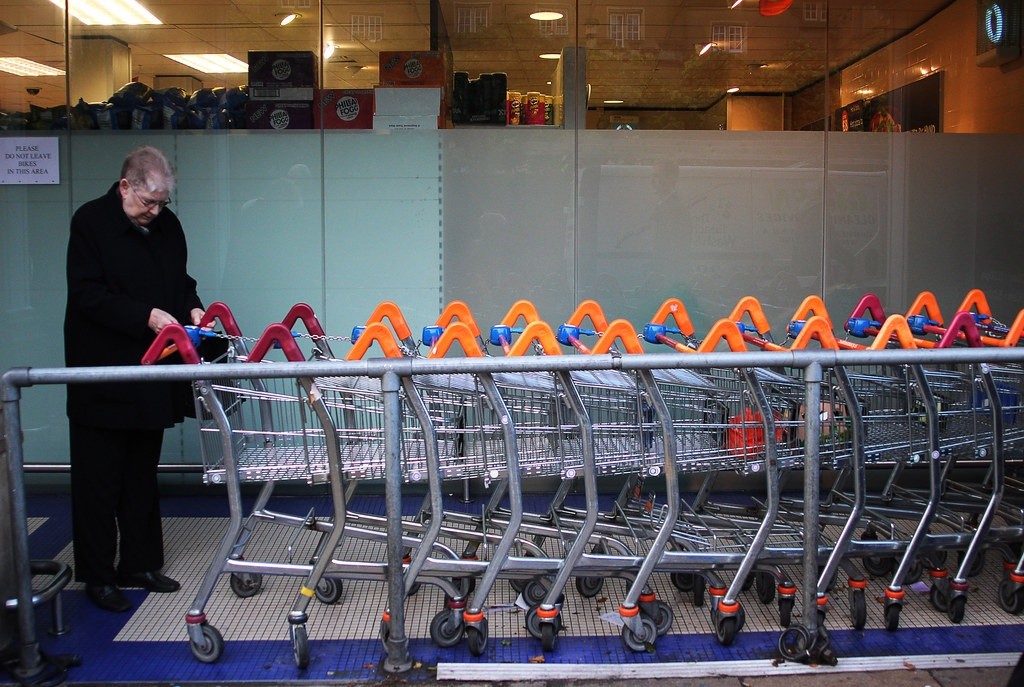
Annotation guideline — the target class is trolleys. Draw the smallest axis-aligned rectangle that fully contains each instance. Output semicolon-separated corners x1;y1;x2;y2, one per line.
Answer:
138;288;1023;669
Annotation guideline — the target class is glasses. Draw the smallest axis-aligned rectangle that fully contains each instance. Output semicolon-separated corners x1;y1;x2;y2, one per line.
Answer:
127;180;171;207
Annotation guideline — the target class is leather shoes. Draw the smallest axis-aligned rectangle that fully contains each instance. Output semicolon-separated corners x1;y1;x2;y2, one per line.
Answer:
118;570;180;592
84;583;131;614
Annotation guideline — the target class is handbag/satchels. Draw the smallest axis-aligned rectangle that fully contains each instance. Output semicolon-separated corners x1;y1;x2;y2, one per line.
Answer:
178;329;247;421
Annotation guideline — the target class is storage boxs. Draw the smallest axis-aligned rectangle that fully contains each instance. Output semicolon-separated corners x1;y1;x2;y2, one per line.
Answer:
244;49;450;129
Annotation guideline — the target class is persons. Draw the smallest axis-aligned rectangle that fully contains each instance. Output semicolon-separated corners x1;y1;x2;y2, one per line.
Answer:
64;145;217;613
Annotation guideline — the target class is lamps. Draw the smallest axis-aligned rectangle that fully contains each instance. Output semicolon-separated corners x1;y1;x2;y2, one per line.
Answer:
275;13;302;27
346;66;368;77
693;43;712;56
